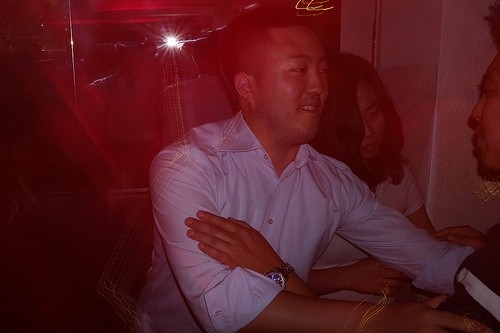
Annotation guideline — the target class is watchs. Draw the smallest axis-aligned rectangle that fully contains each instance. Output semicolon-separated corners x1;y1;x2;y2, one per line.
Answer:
259;262;295;292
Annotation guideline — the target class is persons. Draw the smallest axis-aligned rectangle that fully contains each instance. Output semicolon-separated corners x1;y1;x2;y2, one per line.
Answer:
184;1;500;333
132;6;476;333
308;53;435;296
161;24;233;142
